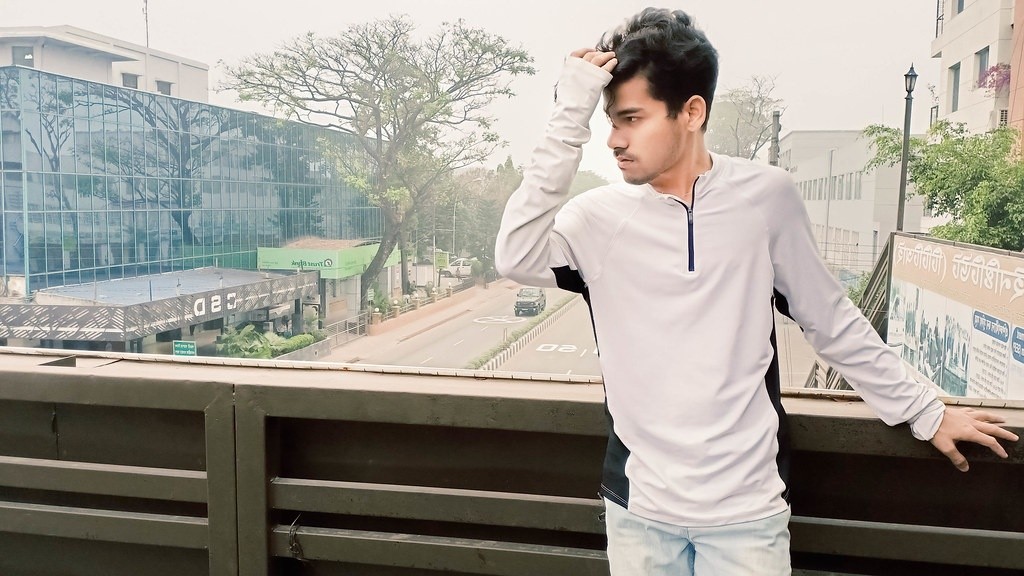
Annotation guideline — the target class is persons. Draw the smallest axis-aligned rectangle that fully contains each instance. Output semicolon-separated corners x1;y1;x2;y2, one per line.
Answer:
494;7;1018;576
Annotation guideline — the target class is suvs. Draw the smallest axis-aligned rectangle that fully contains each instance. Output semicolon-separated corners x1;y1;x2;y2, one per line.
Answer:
513;287;546;316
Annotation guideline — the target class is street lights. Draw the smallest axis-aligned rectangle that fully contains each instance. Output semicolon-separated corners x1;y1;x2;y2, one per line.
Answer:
895;62;918;232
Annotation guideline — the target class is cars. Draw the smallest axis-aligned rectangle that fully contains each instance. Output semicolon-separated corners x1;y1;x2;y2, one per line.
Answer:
440;258;472;278
